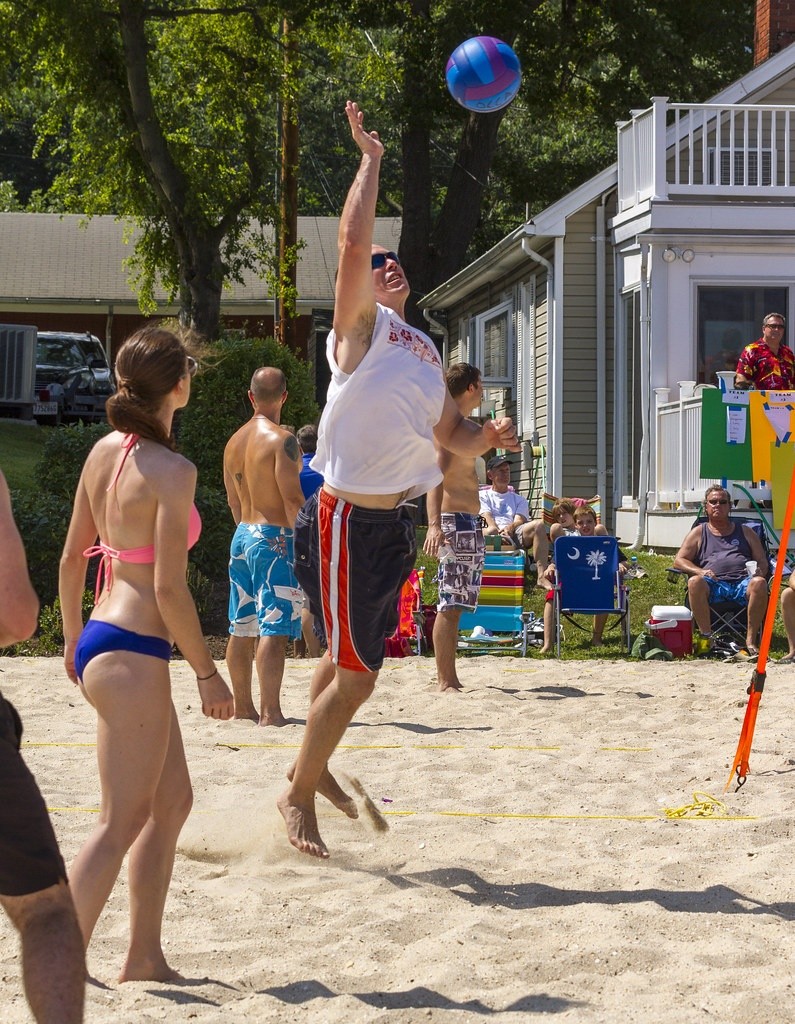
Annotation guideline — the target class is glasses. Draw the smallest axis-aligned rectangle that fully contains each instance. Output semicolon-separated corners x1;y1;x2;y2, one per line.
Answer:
704;499;728;505
371;251;401;269
175;355;199;380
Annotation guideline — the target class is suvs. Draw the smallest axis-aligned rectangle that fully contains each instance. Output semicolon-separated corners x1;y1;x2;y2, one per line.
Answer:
34;332;115;425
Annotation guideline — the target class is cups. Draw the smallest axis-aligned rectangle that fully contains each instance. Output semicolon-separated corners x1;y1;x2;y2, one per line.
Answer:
745;561;758;576
470;626;486;638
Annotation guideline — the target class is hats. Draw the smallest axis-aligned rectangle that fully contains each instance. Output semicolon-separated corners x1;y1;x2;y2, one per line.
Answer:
487;456;514;468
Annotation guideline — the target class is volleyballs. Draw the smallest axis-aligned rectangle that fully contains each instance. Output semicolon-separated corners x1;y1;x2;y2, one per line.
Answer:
446;35;522;114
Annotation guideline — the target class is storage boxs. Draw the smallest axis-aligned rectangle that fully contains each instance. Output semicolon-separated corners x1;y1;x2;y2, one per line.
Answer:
645;605;693;659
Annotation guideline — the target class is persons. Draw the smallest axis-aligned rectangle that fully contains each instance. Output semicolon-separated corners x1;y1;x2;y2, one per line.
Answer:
59;326;235;983
708;328;741;387
478;456;553;591
736;313;795;391
294;424;324;657
223;366;306;727
675;485;768;654
422;363;485;692
540;498;628;652
0;470;85;1024
779;569;795;659
277;100;522;858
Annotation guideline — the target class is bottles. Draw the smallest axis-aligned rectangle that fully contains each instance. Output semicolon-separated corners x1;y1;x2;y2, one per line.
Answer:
417;568;424;590
697;633;710;655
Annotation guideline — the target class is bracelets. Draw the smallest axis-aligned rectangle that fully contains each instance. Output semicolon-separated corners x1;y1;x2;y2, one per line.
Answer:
197;668;217;680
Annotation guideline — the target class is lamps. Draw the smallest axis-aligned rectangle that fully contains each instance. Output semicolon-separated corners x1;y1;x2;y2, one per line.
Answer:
663;244;696;263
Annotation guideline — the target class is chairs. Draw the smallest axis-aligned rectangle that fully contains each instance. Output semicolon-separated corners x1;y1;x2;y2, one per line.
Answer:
46;352;65;364
385;483;774;663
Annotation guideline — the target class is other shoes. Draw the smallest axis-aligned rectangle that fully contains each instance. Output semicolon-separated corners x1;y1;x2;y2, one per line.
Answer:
746;646;759;662
693;638;711;658
